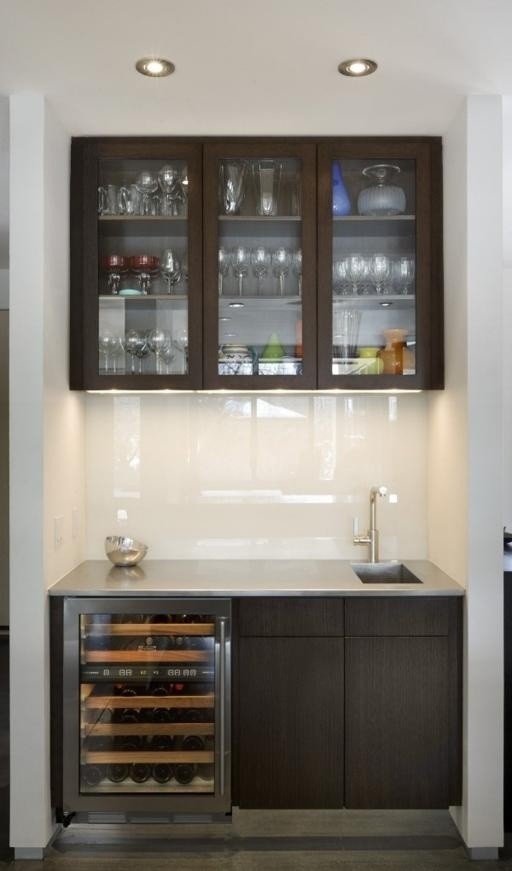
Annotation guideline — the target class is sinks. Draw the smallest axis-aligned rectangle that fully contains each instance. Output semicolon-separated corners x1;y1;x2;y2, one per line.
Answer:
346;558;425;586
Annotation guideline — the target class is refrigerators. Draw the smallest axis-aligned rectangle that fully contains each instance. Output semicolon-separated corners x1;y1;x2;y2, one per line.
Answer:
59;597;234;823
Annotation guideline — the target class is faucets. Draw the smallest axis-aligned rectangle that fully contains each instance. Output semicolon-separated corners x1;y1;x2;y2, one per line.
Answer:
353;484;388;564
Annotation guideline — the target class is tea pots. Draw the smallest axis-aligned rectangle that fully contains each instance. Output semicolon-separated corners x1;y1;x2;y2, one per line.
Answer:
220;344;257;370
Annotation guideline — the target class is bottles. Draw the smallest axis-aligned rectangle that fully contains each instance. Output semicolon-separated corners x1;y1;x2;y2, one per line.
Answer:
79;610;213;788
261;332;287;361
296;318;303;359
331;161;351;216
379;329;415;376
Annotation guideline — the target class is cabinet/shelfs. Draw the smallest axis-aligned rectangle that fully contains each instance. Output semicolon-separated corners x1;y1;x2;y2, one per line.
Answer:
338;583;468;819
48;585;241;831
229;589;350;819
199;132;322;397
65;128;208;396
309;125;449;400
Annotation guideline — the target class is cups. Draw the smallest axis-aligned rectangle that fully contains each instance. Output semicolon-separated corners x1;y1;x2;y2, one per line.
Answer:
332;308;363;378
220;158;251;215
249;160;284;214
99;183;146;216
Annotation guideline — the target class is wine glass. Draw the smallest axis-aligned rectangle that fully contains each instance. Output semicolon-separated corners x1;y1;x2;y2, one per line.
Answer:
127;255;158;295
101;254;130;296
138;164;187;216
99;331;191;375
218;245;304;297
158;248;182;295
333;253;414;298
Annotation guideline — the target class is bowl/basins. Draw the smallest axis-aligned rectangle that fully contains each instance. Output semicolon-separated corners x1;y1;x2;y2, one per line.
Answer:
356;347;381;358
104;535;148;569
